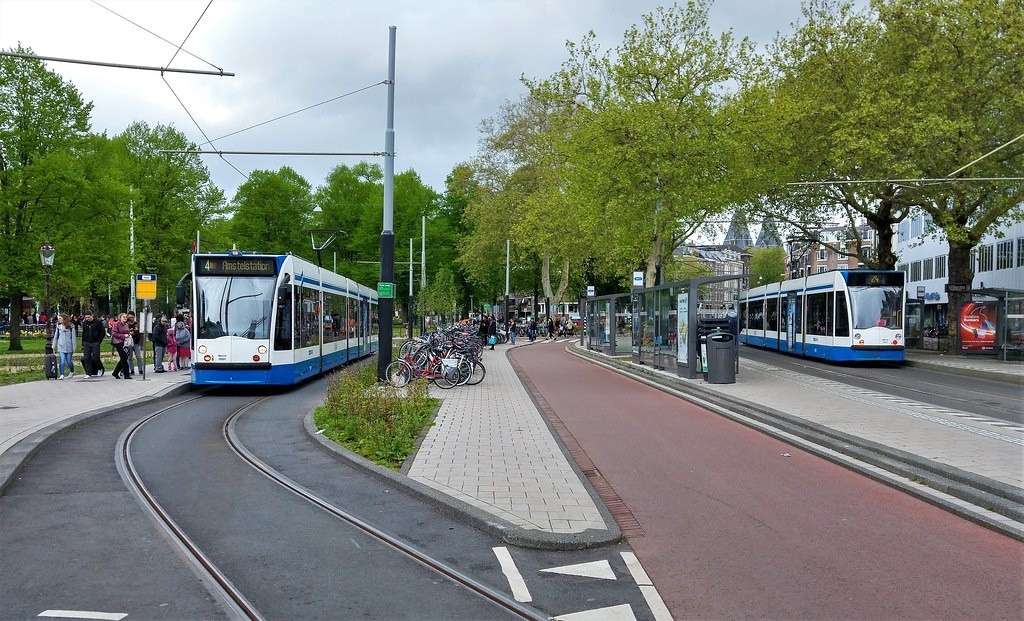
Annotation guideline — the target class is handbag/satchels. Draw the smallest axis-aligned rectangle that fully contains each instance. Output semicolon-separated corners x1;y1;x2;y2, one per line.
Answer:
489;334;496;344
147;333;159;342
123;334;134;347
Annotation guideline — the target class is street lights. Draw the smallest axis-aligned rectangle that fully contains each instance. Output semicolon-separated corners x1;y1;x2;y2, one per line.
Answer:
37;240;57;353
470;295;474;313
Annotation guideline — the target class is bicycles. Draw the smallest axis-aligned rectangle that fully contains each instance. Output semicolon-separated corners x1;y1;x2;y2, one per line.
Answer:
386;321;486;390
487;330;509;343
564;326;585;338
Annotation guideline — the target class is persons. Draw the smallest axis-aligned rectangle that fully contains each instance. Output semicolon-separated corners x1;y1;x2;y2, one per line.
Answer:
3;304;192;380
297;305;376;338
471;308;573;350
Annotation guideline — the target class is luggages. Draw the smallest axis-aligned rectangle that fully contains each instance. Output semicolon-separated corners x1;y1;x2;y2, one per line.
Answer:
80;355;104;374
44;349;57;380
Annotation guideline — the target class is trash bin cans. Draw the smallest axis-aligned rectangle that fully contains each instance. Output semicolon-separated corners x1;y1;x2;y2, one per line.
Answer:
698;317;733;382
707;332;737;384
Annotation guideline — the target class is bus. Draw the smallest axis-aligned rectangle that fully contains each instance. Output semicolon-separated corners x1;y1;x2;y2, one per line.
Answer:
175;228;379;385
738;239;906;365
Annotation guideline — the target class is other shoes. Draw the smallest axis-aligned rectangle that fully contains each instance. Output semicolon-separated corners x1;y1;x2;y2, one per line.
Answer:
124;376;132;379
68;371;75;378
489;347;494;350
112;373;121;379
139;370;142;374
130;370;134;374
155;370;167;373
84;375;91;379
97;368;103;377
179;367;190;370
59;374;64;380
